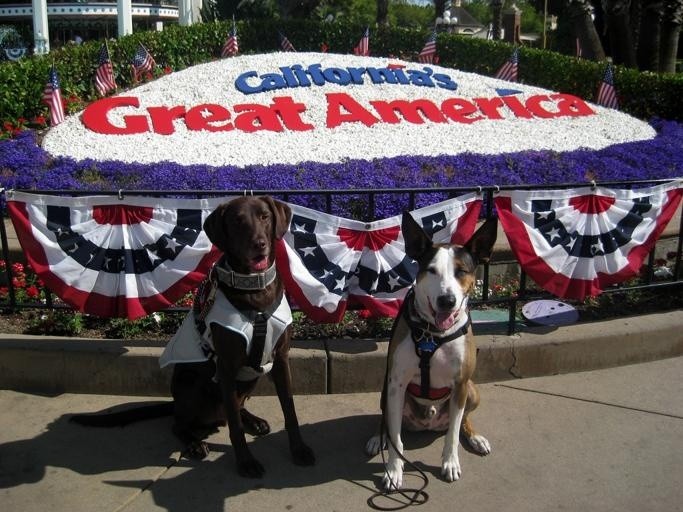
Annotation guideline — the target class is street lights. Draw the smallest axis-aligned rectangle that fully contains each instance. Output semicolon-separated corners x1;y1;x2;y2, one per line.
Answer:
435;10;457;35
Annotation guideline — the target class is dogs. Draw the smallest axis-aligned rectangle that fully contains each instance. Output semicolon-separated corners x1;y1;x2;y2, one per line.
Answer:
365;209;502;492
67;195;318;479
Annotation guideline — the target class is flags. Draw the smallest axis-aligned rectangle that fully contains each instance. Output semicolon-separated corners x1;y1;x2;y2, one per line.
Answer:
128;39;164;78
592;61;619;110
213;12;239;58
93;31;117;98
350;23;368;55
494;42;517;82
274;28;296;52
40;56;66;127
417;24;436;64
